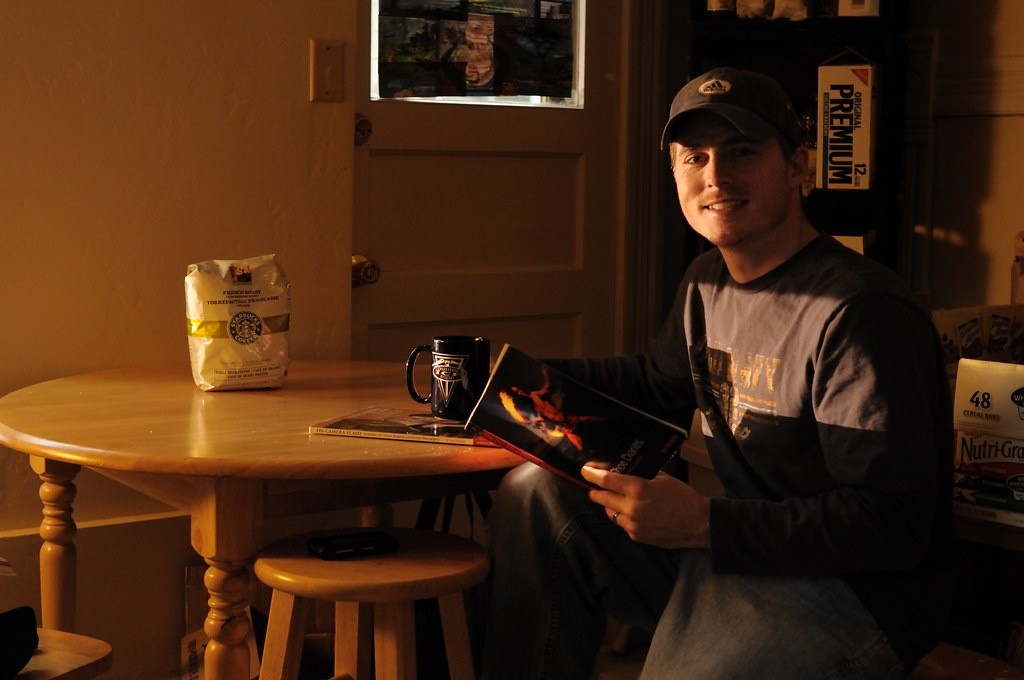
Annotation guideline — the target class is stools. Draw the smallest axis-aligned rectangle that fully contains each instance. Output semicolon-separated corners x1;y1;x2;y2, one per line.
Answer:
17;627;113;680
251;526;492;680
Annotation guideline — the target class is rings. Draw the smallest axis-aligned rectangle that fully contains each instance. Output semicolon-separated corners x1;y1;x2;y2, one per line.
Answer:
613;512;619;524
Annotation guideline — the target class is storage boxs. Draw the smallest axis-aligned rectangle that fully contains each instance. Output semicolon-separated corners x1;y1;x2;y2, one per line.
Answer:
180;564;334;680
952;358;1024;528
816;47;878;190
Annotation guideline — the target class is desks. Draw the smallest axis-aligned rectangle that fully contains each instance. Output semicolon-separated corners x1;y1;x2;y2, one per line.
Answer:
0;359;534;680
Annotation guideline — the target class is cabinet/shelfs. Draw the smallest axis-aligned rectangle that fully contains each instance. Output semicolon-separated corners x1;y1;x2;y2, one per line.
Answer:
681;31;908;336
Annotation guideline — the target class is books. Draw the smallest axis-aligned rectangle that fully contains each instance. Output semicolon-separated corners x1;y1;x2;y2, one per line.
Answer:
465;344;689;496
309;406;502;448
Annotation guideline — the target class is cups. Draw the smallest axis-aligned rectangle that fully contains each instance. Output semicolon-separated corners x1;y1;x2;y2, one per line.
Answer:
405;335;491;421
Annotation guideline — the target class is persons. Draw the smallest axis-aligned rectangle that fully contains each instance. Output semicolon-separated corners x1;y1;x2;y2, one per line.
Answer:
511;365;603;451
486;68;954;680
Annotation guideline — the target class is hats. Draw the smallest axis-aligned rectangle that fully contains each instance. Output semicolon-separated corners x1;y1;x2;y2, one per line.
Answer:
660;68;797;157
